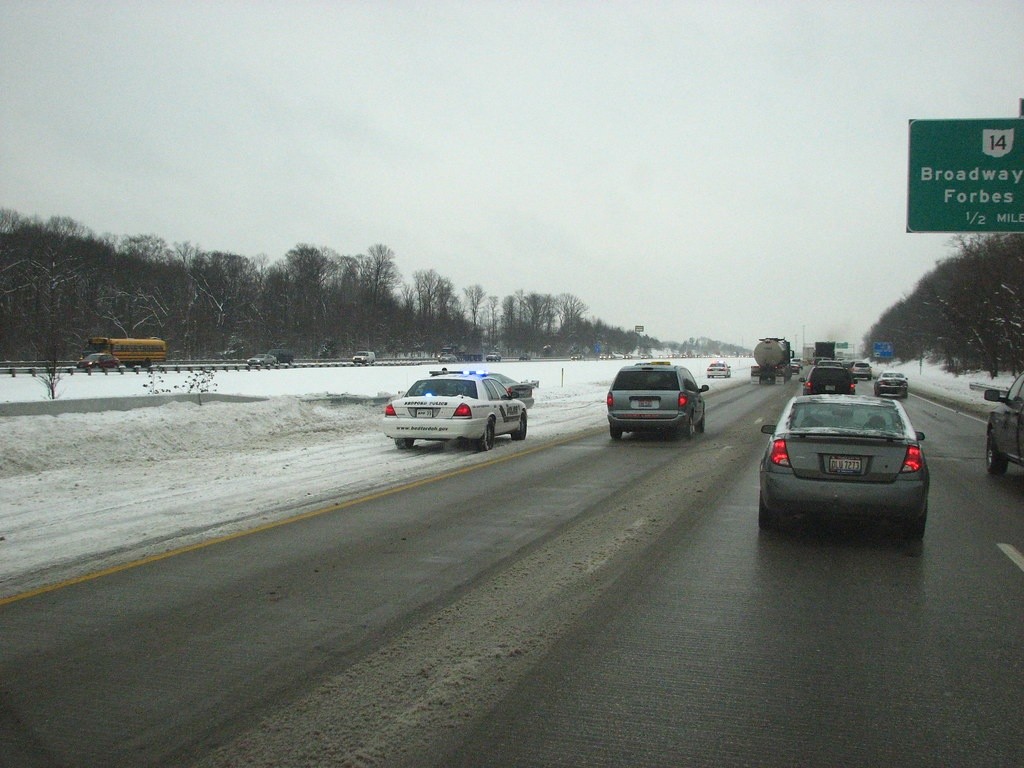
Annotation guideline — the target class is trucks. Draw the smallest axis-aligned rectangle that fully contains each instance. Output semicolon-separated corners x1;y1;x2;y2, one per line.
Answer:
812;341;835;357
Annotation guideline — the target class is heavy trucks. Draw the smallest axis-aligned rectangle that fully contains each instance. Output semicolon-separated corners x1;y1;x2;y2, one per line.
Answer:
441;338;483;361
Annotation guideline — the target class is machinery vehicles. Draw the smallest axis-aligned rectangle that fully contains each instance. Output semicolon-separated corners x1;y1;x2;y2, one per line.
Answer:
750;338;794;385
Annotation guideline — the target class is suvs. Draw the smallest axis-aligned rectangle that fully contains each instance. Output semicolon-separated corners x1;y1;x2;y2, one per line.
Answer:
798;365;858;395
486;352;501;362
606;361;709;439
852;363;872;380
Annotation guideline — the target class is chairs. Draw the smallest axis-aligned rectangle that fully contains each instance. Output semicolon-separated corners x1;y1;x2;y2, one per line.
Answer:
863;416;886;429
799;415;824;427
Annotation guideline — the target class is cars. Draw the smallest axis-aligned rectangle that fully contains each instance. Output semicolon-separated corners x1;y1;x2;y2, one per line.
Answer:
873;372;908;398
571;354;584;360
387;371;534;410
268;349;294;364
383;371;528;451
983;371;1024;475
791;359;804;370
706;361;731;378
247;354;277;366
440;354;457;361
77;353;120;368
791;362;803;374
813;357;862;368
600;354;653;359
519;353;530;361
351;351;376;364
759;394;929;540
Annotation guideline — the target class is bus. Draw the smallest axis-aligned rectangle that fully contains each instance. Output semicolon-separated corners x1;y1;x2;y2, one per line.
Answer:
79;337;166;368
